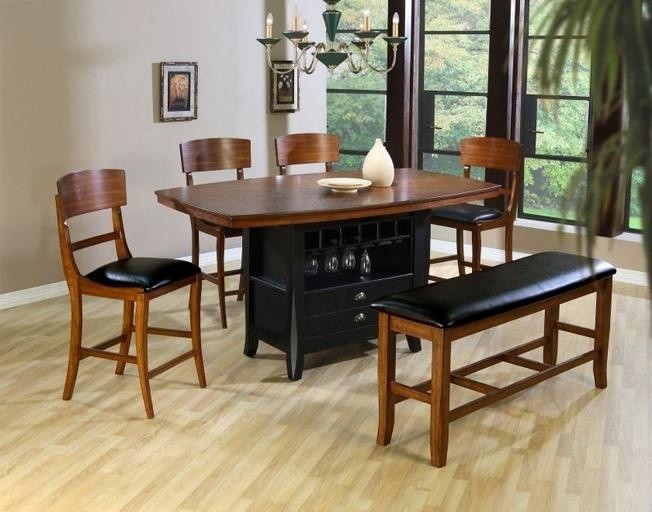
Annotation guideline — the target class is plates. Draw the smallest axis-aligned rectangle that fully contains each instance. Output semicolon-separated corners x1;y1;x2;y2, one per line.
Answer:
318;178;371;193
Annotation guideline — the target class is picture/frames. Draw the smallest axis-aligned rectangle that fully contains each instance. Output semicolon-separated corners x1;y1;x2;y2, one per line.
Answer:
271;60;300;113
160;62;198;122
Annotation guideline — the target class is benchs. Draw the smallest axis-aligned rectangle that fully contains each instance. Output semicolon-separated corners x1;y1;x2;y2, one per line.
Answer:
369;251;616;467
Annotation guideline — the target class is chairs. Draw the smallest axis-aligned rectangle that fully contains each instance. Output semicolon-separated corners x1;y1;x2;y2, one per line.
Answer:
428;137;525;283
180;137;252;327
55;169;208;418
274;133;340;175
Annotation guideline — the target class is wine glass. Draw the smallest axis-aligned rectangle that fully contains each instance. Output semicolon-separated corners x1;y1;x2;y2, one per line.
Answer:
305;248;372;276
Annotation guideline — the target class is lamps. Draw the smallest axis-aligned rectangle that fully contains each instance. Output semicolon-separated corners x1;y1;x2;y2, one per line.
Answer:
257;0;408;81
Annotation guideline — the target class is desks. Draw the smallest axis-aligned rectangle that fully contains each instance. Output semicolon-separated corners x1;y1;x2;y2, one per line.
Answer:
155;168;503;380
